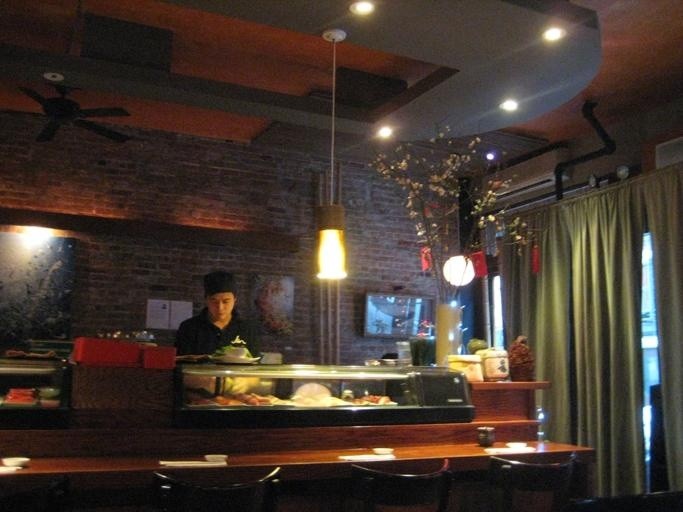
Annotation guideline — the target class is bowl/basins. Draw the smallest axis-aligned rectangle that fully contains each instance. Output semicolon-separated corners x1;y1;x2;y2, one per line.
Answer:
223;347;248;357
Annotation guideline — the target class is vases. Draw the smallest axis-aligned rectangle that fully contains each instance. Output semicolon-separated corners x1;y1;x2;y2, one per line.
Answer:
435;301;464;367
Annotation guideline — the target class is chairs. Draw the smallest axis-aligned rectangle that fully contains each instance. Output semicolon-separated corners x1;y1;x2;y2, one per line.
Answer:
562;489;683;512
488;454;581;512
0;474;77;512
149;467;283;512
345;458;454;512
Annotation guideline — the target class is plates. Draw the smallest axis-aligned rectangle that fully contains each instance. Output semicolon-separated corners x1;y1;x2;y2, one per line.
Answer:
208;354;261;365
507;442;527;448
378;358;412;365
373;447;393;455
1;457;30;467
206;455;228;463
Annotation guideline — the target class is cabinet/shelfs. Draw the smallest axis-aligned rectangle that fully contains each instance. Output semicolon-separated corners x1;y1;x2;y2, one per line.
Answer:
0;358;73;430
183;358;476;426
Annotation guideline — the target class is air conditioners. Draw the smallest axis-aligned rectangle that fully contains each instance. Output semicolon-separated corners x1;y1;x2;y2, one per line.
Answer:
480;148;574;202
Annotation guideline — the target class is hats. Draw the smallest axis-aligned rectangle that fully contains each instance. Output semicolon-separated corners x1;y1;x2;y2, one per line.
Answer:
204;272;233;293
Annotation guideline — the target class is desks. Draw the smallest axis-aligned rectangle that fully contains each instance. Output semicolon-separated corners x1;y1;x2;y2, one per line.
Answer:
0;440;596;490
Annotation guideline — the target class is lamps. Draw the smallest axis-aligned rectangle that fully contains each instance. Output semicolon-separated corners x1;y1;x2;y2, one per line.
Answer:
317;29;349;281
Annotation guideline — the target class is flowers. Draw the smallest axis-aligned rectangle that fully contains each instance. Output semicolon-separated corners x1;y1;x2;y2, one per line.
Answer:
368;122;547;302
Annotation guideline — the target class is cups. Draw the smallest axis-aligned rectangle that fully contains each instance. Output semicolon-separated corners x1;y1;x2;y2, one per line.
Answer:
475;427;496;446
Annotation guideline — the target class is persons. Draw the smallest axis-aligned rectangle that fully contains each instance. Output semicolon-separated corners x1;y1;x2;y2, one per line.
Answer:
174;270;267;358
